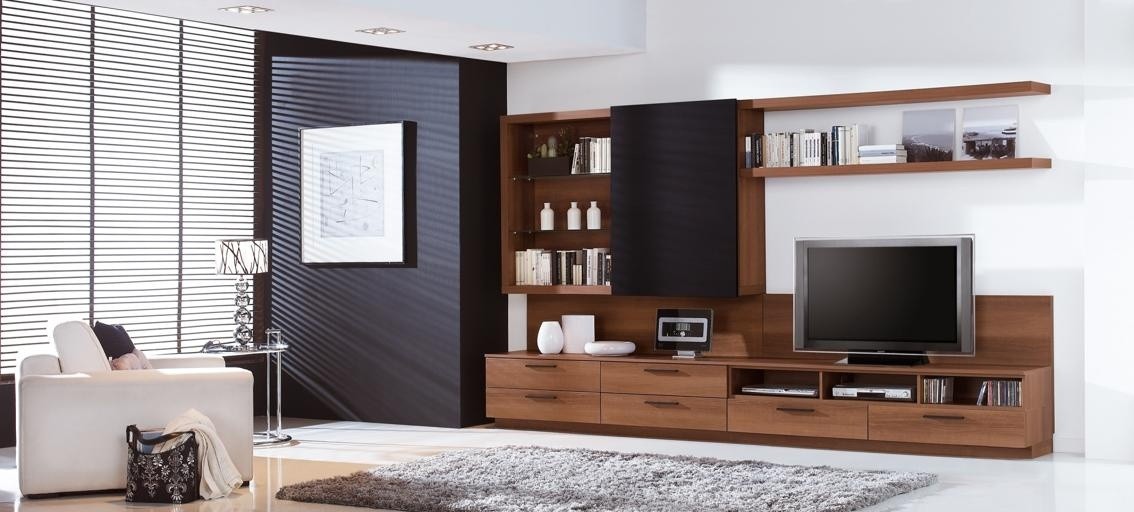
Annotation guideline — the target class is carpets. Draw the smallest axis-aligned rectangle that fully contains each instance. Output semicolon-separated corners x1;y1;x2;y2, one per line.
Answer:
274;443;937;511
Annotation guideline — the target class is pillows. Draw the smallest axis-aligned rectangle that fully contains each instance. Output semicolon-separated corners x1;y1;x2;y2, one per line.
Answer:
92;320;152;372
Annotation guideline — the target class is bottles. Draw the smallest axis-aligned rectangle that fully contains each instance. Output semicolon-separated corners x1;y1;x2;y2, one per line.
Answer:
539;200;556;230
567;201;583;231
585;200;601;231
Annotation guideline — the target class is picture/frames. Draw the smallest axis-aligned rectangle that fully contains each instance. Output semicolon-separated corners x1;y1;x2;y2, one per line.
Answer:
297;120;415;268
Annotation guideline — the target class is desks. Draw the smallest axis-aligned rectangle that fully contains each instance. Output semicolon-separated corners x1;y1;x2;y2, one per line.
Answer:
201;346;293;444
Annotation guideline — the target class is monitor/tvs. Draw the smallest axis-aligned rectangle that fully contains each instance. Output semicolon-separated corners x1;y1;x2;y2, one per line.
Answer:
792;233;976;364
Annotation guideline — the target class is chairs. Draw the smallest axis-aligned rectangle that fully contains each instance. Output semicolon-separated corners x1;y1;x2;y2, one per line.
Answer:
16;347;255;499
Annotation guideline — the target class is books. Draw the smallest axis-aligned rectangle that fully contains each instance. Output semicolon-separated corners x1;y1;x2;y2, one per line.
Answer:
571;136;611;175
976;380;1022;406
923;377;954;404
744;124;907;168
515;248;611;286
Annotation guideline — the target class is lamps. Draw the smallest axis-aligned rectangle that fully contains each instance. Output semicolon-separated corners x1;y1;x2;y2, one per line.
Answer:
214;238;268;348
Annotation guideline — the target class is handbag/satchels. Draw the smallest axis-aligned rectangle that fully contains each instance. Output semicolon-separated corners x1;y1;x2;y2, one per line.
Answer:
126;424;200;503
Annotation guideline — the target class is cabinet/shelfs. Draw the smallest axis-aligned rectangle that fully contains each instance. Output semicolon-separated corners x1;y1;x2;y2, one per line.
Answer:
498;98;767;298
483;346;1055;460
733;78;1053;181
